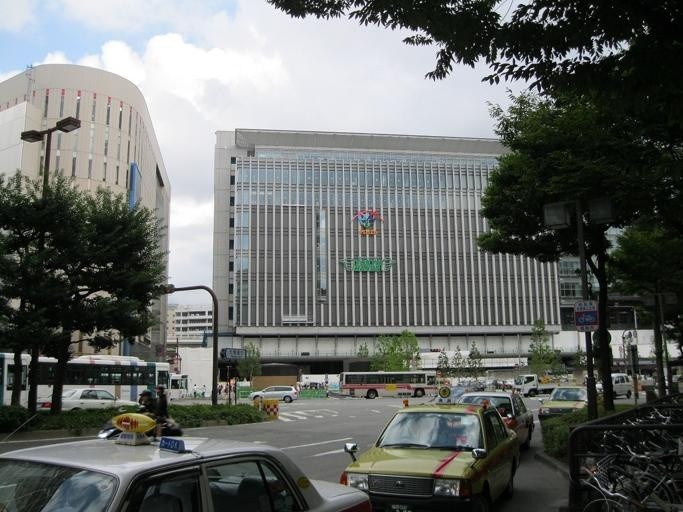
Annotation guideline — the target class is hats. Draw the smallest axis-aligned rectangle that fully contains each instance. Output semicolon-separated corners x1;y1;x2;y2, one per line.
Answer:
154;385;164;392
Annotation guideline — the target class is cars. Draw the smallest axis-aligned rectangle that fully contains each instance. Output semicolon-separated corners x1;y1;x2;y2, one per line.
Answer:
339;390;536;512
454;379;513;390
537;374;682;428
0;412;372;511
249;385;299;403
37;388;145;411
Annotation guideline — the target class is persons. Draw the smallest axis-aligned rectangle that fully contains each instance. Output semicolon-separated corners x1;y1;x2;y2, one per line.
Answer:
627;371;645;382
295;377;306;395
193;376;239;406
325;381;329;397
512;388;523;398
479;382;484;391
502;380;505;391
136;381;179;418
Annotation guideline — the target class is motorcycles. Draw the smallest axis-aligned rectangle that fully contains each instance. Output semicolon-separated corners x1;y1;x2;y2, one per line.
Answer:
97;415;184;438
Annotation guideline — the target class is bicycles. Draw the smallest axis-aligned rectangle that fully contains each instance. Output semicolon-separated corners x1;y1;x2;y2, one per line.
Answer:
575;395;683;512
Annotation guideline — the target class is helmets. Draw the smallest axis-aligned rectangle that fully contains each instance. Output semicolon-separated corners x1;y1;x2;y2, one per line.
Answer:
138;389;153;396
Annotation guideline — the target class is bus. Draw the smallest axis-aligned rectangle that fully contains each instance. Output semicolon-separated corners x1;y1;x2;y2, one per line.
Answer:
339;370;437;399
0;352;187;405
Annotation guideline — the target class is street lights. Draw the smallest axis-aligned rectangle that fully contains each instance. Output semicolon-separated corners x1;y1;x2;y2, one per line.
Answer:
21;116;81;410
539;196;617;420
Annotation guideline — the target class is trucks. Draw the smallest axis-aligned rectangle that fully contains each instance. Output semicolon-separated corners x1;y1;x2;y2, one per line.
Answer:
514;374;560;397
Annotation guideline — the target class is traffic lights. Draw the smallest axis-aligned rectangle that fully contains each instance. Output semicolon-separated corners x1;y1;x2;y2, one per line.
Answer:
153;285;175;295
